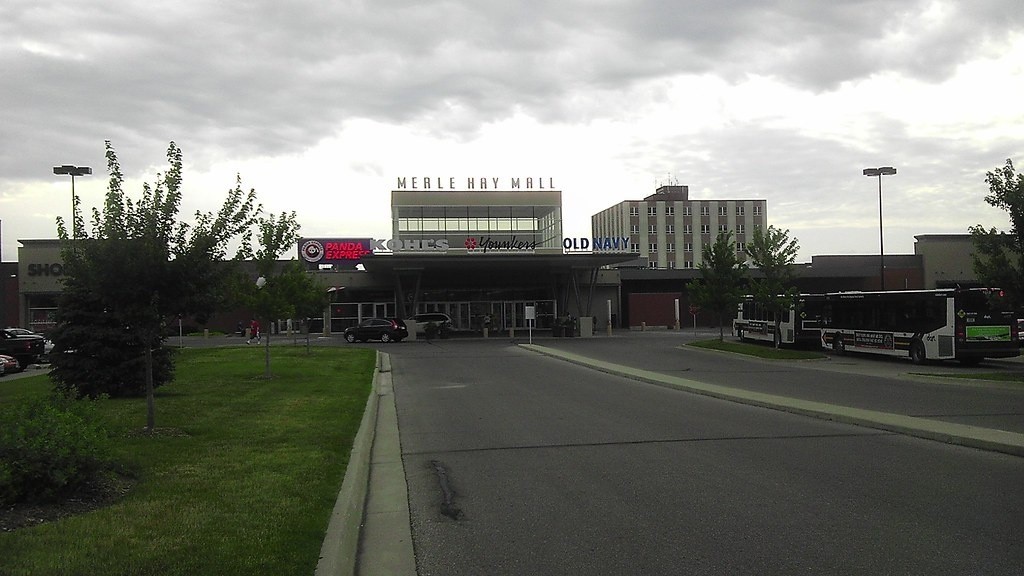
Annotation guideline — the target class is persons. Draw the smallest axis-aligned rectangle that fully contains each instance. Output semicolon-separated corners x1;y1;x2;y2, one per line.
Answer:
245;318;261;345
611;313;616;329
237;321;245;336
483;313;491;335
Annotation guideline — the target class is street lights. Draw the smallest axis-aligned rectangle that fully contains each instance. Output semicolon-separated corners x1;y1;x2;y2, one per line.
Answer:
53;165;93;252
863;166;898;290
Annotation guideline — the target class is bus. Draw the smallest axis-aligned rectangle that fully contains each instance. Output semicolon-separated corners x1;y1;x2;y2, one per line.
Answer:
731;293;830;349
820;285;1021;366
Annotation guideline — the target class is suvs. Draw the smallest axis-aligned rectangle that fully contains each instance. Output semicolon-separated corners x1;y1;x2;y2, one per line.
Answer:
344;318;409;344
409;314;454;335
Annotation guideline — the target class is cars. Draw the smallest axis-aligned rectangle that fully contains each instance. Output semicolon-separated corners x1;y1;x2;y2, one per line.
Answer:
0;328;58;374
